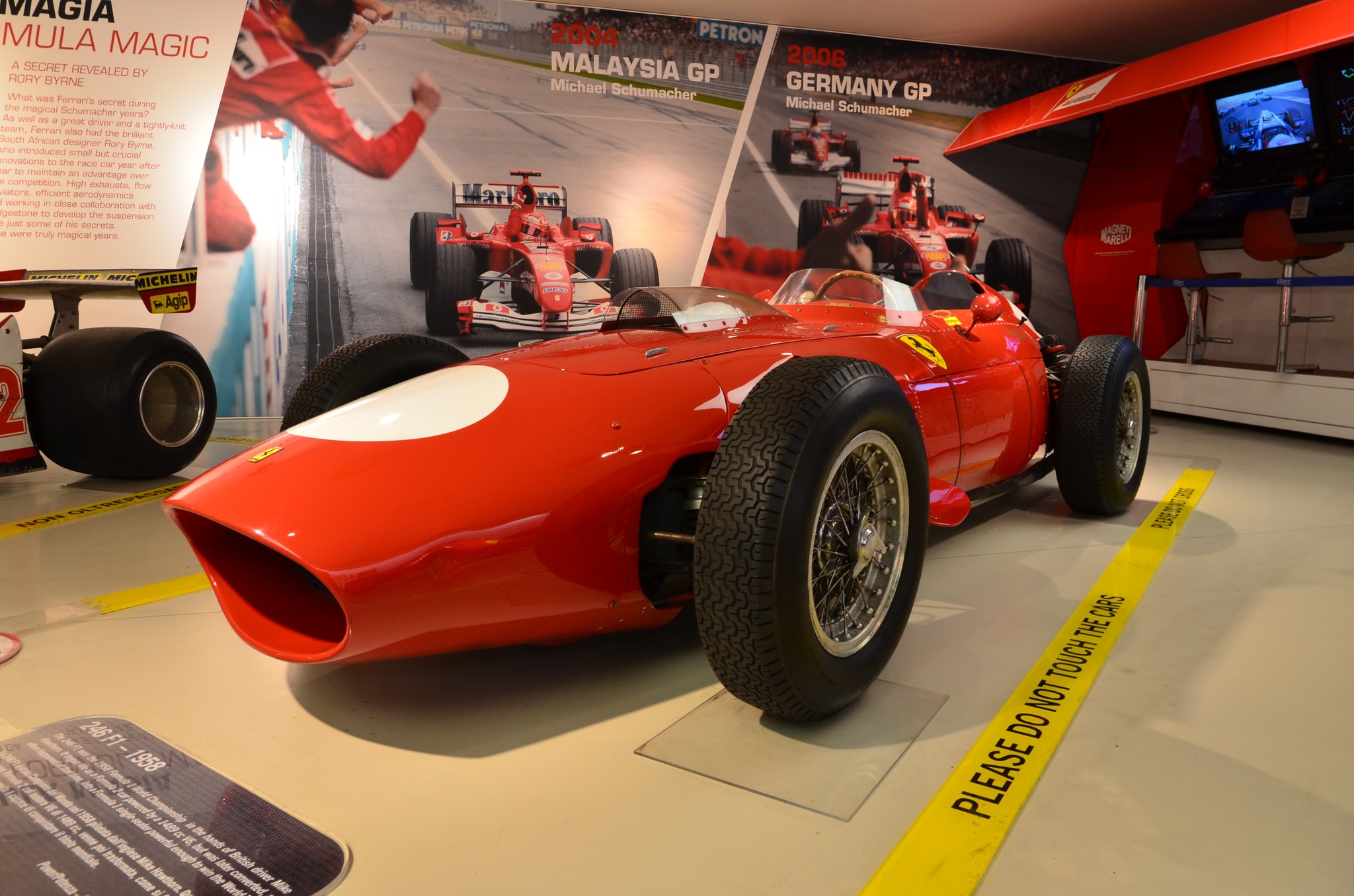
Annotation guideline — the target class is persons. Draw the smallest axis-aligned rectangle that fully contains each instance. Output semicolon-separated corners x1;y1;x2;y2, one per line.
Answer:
205;0;1047;274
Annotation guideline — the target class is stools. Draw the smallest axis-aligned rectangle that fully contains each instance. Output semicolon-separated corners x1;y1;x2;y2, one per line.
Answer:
1243;209;1344;374
1156;243;1242;366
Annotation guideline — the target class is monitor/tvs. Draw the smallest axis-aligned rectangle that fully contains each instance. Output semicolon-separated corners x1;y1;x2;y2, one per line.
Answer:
1211;74;1319;163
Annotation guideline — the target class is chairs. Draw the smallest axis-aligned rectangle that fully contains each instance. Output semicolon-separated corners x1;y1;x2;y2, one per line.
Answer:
910;271;979;310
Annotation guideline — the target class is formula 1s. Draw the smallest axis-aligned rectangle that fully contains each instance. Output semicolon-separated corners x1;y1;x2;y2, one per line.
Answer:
797;156;1033;316
162;264;1151;730
771;110;861;175
409;170;660;344
0;268;219;485
